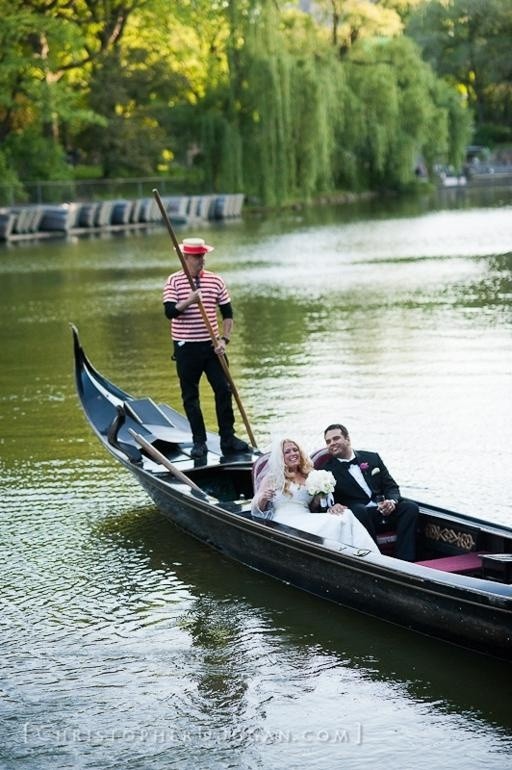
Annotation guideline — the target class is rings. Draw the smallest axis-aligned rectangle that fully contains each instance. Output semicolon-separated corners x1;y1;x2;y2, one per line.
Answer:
389;506;391;508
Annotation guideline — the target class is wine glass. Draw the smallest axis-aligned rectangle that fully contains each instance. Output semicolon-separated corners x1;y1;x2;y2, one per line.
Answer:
267;478;278;502
375;494;389;525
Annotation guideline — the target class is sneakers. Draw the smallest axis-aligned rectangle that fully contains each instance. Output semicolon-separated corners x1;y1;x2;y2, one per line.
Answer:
220;435;248;452
191;441;209;458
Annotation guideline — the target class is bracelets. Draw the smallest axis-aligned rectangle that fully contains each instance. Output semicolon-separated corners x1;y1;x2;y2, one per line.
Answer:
221;335;231;344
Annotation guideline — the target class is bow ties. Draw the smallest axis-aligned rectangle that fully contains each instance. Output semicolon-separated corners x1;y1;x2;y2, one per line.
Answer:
340;457;359;469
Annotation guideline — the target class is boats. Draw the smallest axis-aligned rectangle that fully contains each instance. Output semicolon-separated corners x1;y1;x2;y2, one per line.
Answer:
67;321;512;664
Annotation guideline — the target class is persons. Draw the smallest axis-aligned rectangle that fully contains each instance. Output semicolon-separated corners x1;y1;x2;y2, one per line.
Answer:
320;423;420;563
161;235;249;460
251;437;383;556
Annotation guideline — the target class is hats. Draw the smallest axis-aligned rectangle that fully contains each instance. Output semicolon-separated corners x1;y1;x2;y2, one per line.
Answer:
172;237;214;254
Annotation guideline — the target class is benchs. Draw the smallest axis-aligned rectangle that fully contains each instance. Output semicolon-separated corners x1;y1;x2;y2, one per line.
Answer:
412;546;494;572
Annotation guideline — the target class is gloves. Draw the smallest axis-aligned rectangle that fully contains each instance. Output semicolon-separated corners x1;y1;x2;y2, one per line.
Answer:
211;335;231;356
